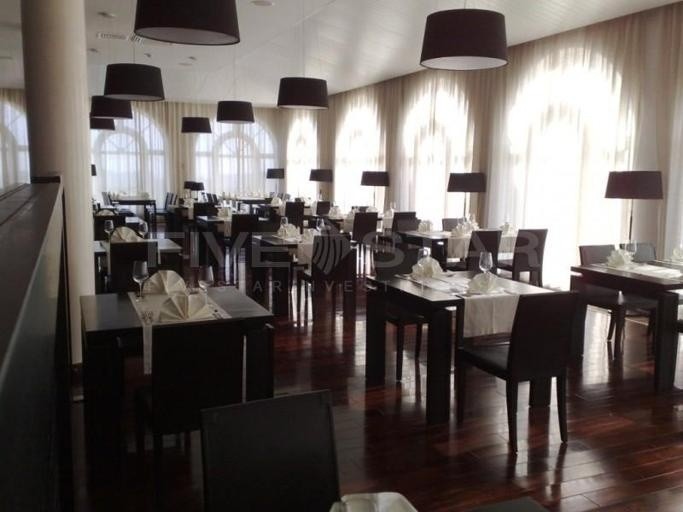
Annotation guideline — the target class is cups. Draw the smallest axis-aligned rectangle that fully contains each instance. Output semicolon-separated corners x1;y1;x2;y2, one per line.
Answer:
673;238;683;260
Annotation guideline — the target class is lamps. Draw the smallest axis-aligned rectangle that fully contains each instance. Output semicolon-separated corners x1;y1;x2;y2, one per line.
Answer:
445;171;488;223
359;169;390;210
601;169;666;262
87;0;510;137
190;181;204;199
182;180;194;197
308;168;334;200
266;167;284;194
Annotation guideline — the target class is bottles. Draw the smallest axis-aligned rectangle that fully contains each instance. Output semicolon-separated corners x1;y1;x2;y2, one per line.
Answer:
301;218;309;239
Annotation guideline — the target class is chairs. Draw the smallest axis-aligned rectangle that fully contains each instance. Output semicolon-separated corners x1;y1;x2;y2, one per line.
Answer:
78;192;682;511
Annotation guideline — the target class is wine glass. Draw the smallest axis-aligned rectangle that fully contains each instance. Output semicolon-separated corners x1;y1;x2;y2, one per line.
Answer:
477;251;493;273
389;200;396;216
132;260;148;301
455;213;477;227
277;216;289;231
103;219;113;241
416;248;430;289
626;239;637;256
197;264;214;309
315;216;325;234
137;222;148;238
